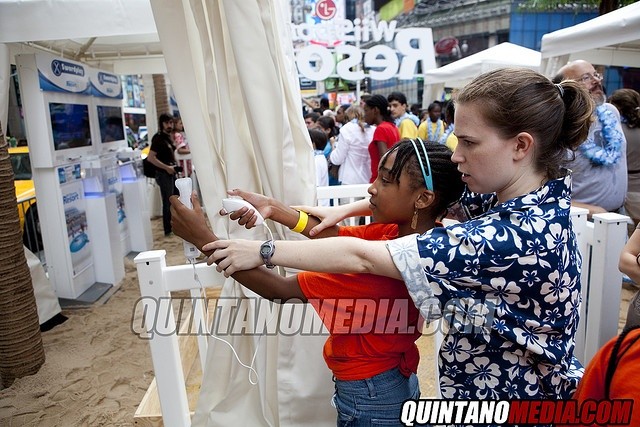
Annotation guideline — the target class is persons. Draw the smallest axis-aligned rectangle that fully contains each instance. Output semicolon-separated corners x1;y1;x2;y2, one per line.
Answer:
147;113;179;243
305;113;321;128
169;137;467;426
555;59;629;212
572;290;640;425
306;128;334;206
330;106;376;184
319;97;336;120
102;117;124;142
362;94;400;172
410;104;424;125
172;117;191;175
606;88;639;284
201;67;595;425
438;99;458;153
313;108;323;116
419;102;446;141
387;93;419;138
336;104;351;126
315;116;338;158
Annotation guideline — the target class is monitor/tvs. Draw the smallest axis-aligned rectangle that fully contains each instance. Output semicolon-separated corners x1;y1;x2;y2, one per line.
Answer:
49;103;92;150
96;105;125;142
125;113;146;134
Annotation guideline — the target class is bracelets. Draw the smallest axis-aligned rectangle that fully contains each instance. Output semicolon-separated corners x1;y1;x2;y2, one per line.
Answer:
290;210;309;233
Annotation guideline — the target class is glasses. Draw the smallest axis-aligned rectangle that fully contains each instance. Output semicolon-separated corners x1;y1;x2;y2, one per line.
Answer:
576;71;604;83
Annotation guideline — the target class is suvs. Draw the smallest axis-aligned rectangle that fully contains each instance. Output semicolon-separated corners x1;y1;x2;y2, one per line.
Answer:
8;146;43;253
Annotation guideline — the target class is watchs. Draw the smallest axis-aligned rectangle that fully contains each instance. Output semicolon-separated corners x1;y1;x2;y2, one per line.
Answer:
259;239;276;269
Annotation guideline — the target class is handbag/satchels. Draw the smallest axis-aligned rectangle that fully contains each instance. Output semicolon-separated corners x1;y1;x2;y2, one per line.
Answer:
9;153;31;179
142;132;175;178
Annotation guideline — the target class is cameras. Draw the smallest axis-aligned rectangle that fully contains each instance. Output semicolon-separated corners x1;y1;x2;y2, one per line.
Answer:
174;166;183;172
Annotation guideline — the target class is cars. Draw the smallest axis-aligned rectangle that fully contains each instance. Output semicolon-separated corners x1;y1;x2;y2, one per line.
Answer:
138;126;148;139
126;125;137;142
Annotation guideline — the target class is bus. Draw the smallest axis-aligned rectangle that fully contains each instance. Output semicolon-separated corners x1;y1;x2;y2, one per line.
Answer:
123;107;146;140
